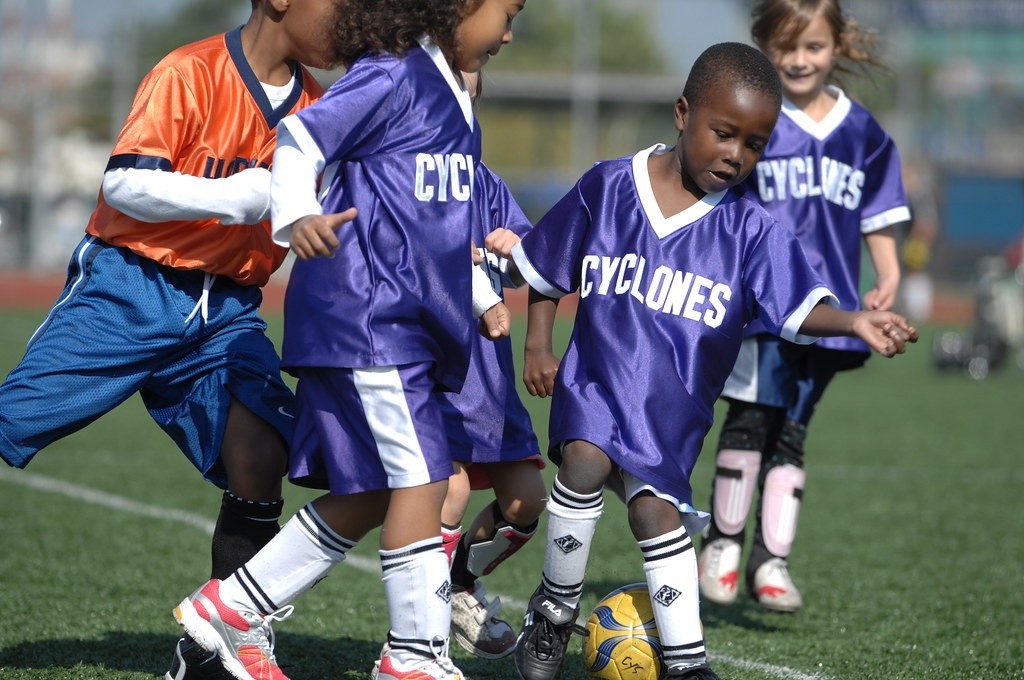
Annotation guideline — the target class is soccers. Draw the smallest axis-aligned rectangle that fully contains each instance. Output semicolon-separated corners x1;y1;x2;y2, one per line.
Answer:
581;581;706;680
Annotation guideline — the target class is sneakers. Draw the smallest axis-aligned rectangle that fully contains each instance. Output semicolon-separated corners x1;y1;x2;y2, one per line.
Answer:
515;584;592;679
165;634;239;680
664;662;719;679
369;629;470;680
171;578;295;680
448;579;518;659
698;521;804;612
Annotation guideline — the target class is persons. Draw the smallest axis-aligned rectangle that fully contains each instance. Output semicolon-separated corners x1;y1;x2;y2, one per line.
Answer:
512;40;917;678
700;0;911;613
440;66;547;659
0;2;346;680
176;2;526;680
